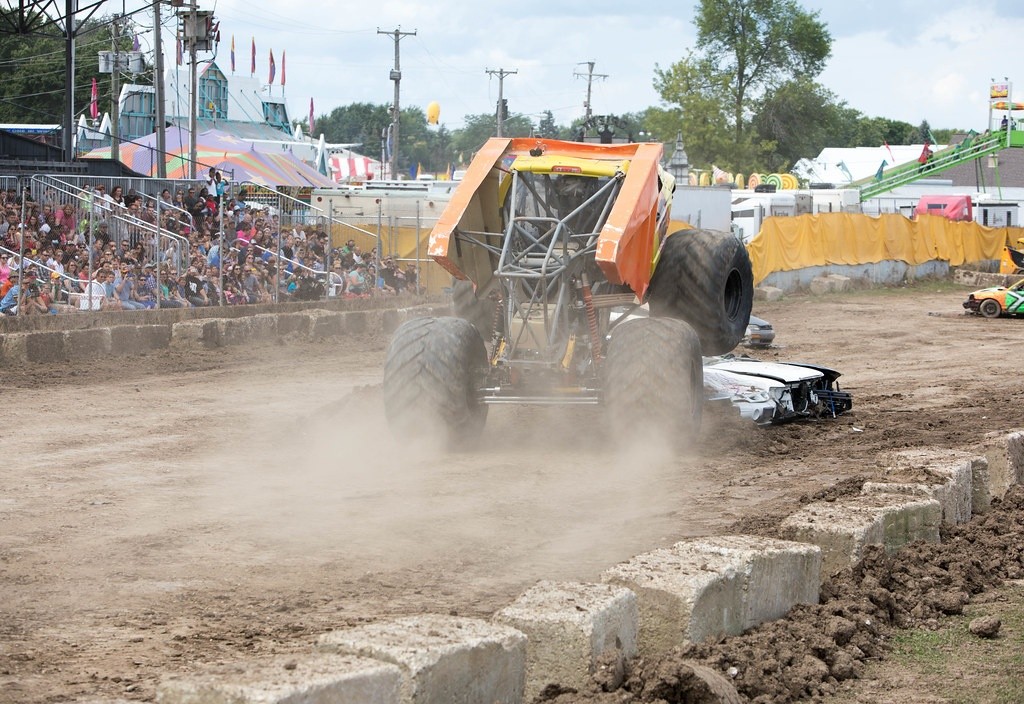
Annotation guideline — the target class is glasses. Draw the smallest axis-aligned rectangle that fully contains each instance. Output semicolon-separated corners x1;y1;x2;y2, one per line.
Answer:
42;254;49;257
31;219;36;220
103;226;107;228
161;273;167;275
246;250;253;252
137;247;142;249
1;257;8;259
105;254;112;256
170;273;177;275
6;207;13;210
24;236;31;238
99;276;107;279
140;279;146;281
204;234;211;236
195;205;202;207
154;271;157;273
247;256;254;260
100;188;105;190
245;270;251;272
23;282;30;284
10;229;16;230
235;268;241;270
44;287;50;289
109;245;116;247
70;264;76;266
84;186;88;188
122;272;127;274
122;244;129;247
190;192;195;194
80;247;85;249
296;241;300;243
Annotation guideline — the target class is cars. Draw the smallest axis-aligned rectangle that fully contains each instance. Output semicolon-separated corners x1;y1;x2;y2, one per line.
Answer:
961;277;1024;318
610;305;854;426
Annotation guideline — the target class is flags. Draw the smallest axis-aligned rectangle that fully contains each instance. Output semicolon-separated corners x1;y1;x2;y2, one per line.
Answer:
133;32;140;51
90;78;97;118
281;51;285;85
231;35;235;70
961;129;980;148
835;162;853;182
928;130;938;147
874;160;888;181
883;141;894;162
446;162;455;181
176;29;182;65
251;37;255;72
310;97;314;133
410;159;422;180
269;49;275;84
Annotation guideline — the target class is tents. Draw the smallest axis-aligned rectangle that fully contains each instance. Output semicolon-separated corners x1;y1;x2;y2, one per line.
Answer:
79;125;346;188
328;156;378;183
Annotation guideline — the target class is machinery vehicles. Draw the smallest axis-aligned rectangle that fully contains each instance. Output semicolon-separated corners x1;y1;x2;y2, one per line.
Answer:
381;134;754;470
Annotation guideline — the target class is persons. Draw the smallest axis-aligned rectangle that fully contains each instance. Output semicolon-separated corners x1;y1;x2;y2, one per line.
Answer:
918;142;935;173
983;129;990;149
0;167;421;317
969;133;982;154
953;145;961;160
1001;115;1016;131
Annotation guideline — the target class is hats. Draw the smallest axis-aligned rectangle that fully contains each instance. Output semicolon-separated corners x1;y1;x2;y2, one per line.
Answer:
26;271;38;279
263;204;270;210
233;205;241;211
129;188;137;196
9;271;19;278
121;269;128;273
66;240;74;245
100;222;108;227
51;273;60;279
144;263;154;269
98;185;105;190
